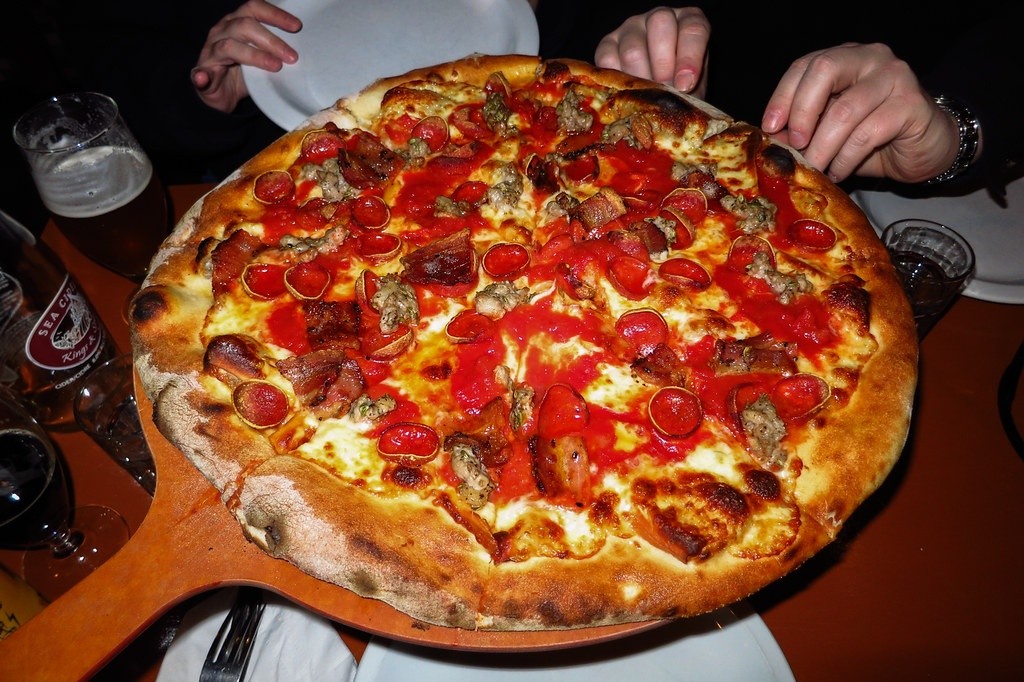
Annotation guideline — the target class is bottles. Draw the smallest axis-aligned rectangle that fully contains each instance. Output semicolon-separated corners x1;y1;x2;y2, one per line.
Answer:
0;210;125;434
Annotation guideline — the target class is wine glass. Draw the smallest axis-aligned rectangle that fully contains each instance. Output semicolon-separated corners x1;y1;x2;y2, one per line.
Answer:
0;384;129;605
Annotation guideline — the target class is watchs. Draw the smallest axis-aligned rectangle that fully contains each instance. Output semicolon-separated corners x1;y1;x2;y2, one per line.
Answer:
922;94;980;184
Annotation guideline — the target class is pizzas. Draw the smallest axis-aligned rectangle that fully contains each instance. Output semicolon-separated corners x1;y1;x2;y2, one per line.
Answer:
128;55;917;633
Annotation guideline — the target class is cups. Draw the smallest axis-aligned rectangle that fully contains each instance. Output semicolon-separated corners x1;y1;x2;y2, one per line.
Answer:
73;352;157;496
14;92;176;286
881;219;977;343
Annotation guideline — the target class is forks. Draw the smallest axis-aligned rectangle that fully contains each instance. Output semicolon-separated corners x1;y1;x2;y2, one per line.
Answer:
199;600;267;682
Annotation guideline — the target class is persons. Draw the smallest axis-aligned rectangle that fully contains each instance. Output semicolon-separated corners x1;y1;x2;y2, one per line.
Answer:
68;1;580;182
595;0;1024;206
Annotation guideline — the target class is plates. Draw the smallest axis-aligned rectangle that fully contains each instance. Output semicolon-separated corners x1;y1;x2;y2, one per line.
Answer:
242;0;540;132
355;600;799;682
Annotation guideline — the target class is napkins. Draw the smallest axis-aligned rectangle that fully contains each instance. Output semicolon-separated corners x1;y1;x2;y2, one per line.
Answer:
152;586;358;682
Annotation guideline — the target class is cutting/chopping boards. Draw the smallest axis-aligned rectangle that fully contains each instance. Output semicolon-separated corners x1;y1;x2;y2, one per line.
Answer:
0;355;676;682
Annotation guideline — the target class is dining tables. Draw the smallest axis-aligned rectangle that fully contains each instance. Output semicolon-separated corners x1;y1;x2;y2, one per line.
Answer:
0;185;1024;682
